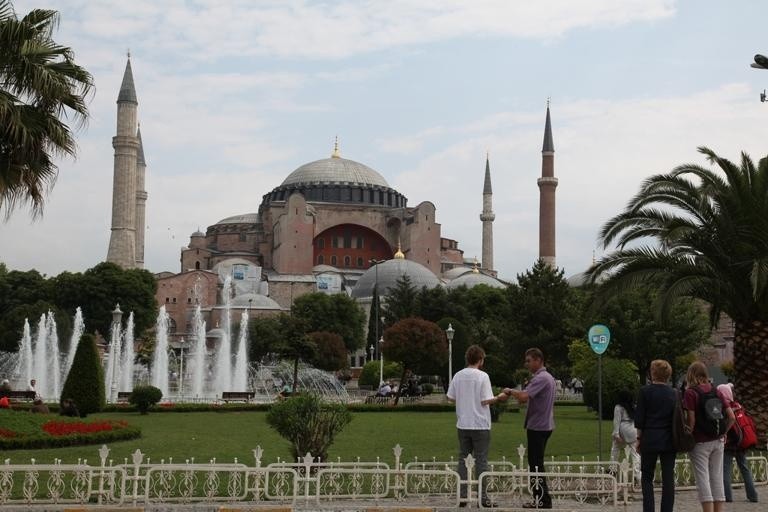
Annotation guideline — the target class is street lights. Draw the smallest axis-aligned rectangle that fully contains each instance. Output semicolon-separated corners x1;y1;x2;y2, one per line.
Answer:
370;335;384;390
110;303;123;404
445;323;456;381
180;336;185;392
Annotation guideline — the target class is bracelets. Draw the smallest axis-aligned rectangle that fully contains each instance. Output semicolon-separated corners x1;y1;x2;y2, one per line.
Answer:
508;388;513;395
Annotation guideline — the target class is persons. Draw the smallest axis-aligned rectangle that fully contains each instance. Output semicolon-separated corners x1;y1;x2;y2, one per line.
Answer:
270;380;290;401
25;378;39;399
31;399;49;414
603;385;641;486
500;347;558;509
444;344;507;508
631;358;682;512
712;381;759;504
401;379;413;397
390;384;398;393
58;399;80;418
373;381;390;399
677;360;735;512
0;379;11;401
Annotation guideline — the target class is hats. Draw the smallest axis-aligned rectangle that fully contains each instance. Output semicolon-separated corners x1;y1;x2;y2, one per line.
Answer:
716;383;735;402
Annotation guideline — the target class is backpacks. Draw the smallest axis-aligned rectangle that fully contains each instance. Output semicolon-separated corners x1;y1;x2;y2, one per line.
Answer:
686;384;729;442
728;404;758;452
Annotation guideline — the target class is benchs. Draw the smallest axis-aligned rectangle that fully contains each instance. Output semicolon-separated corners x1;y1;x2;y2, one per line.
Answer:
280;392;303;401
367;395;421;406
218;392;256;404
7;391;36;403
117;392;134;404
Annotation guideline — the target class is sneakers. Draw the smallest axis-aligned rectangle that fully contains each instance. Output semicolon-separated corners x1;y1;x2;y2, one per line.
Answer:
726;496;759;503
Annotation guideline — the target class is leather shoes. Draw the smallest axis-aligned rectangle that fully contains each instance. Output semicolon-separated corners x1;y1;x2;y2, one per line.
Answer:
460;497;554;509
